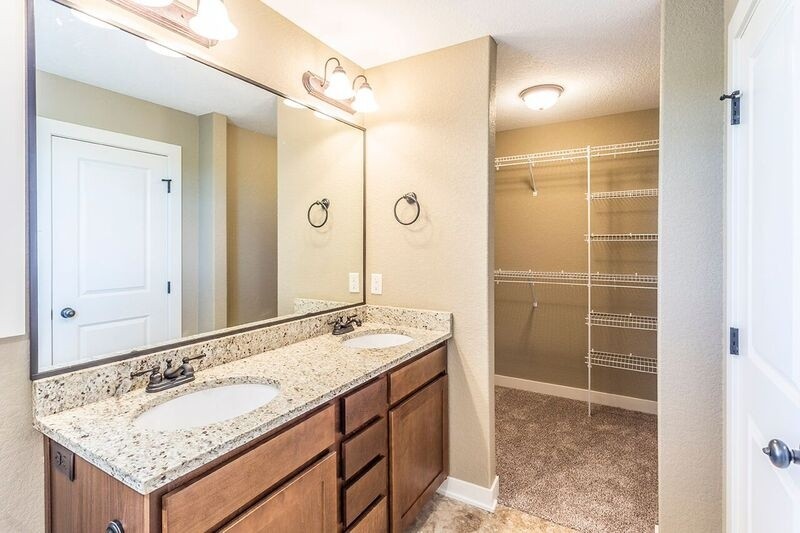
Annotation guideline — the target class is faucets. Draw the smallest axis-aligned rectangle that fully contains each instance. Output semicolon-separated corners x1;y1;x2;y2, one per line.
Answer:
131;354;207;393
328;314;361;335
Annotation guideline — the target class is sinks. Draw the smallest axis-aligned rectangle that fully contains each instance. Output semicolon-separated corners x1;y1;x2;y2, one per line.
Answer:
339;328;416;349
130;376;282;427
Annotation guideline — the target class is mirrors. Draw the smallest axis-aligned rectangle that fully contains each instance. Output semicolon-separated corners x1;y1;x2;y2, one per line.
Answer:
25;0;367;380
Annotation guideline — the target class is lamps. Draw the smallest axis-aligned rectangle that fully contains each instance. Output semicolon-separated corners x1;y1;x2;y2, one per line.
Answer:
110;0;237;48
303;57;378;115
518;85;563;113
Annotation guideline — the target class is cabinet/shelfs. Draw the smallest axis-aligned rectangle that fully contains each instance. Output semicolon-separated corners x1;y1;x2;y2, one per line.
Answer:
42;341;449;533
